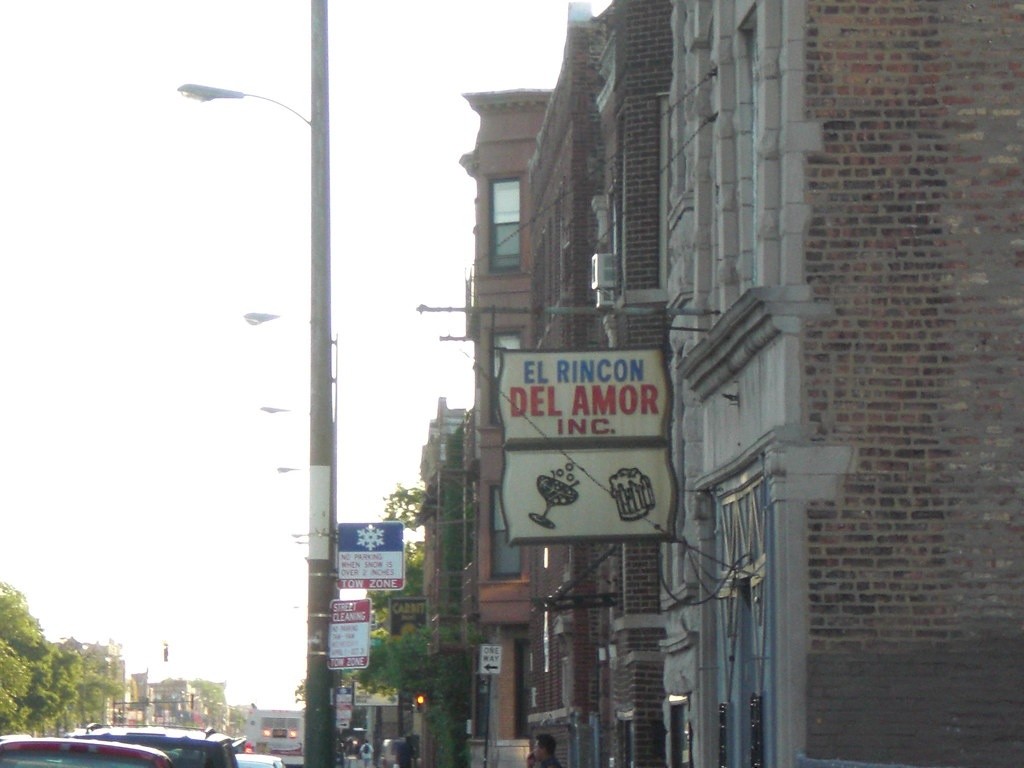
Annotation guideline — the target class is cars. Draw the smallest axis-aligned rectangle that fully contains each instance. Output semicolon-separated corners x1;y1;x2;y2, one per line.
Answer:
234;752;287;768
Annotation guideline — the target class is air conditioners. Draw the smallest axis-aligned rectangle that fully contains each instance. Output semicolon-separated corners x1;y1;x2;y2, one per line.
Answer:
592;253;616;285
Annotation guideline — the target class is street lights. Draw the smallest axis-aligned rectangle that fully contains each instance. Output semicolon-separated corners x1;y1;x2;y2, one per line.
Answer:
170;0;344;768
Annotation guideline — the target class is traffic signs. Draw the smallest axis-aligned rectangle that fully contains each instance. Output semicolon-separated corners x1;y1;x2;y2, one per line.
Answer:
477;643;504;676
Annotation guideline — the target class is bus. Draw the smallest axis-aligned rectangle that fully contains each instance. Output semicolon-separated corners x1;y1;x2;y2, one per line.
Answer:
243;709;306;768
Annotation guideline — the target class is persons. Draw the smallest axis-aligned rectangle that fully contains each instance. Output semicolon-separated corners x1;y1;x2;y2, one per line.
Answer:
360;738;374;768
527;733;561;768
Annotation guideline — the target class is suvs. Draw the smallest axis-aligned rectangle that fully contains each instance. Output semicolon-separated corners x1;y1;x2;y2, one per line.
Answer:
0;734;174;768
60;723;246;768
